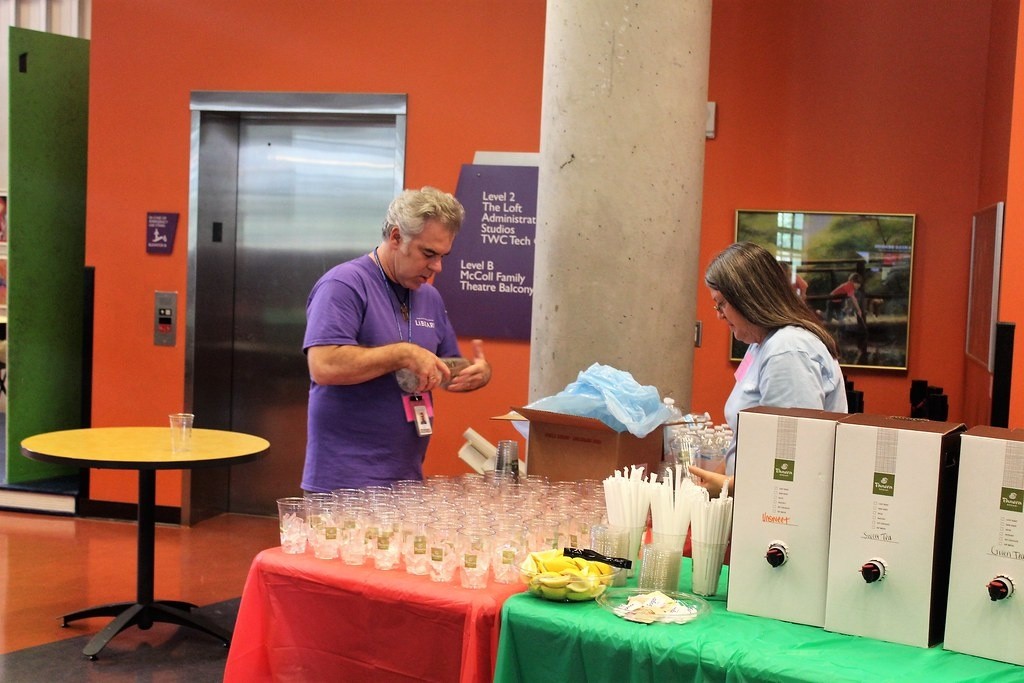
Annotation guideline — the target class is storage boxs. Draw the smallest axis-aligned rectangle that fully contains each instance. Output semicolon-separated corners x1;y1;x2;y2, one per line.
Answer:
490;406;708;485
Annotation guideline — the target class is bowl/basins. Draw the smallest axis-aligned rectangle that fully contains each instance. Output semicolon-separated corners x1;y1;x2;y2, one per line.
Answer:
519;567;622;601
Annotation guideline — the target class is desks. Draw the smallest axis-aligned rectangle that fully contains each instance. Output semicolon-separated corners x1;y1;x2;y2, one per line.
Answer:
221;489;693;682
21;426;270;657
494;546;1024;682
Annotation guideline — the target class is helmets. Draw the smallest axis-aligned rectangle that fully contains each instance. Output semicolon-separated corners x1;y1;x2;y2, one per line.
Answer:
848;271;862;284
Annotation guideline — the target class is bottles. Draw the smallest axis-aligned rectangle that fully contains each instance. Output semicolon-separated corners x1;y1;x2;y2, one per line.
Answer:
638;543;682;591
664;397;733;471
496;440;519;474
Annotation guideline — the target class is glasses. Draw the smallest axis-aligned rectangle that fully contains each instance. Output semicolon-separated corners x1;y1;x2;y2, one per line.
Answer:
713;298;727;313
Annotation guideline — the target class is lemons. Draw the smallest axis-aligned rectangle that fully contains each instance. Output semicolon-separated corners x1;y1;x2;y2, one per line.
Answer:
527;552;616;600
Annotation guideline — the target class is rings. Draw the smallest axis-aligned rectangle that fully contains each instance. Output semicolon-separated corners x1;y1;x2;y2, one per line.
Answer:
431;376;435;379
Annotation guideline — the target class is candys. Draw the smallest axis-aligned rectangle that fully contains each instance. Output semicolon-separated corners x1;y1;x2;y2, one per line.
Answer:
613;590;698;624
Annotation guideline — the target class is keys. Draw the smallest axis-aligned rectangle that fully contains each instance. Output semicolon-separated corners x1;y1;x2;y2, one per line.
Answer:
401;305;408;320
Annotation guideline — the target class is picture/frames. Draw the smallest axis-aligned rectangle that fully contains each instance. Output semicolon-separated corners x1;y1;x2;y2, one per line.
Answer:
726;205;916;378
963;199;1006;373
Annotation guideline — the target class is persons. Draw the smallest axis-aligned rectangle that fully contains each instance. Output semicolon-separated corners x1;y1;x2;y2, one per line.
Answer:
815;273;863;322
300;185;491;511
779;262;807;302
420;413;426;424
688;241;848;500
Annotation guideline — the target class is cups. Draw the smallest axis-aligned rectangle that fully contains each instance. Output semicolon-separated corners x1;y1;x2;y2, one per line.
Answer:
609;520;643;576
396;358;471;393
589;525;630;586
169;413;194;452
689;539;728;595
277;470;607;589
651;530;687;551
668;434;702;486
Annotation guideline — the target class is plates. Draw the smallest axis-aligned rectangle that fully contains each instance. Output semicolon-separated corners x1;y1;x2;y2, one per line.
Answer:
596;588;709;624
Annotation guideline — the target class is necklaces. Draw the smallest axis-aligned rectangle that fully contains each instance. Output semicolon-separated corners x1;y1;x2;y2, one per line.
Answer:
384;271;408;305
